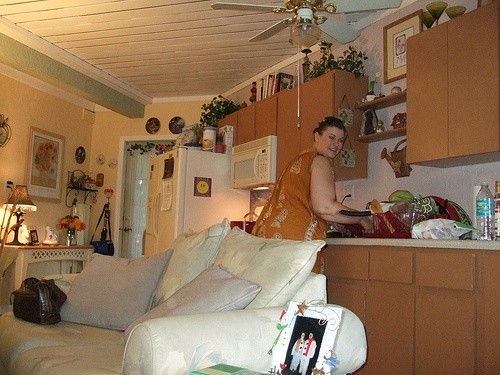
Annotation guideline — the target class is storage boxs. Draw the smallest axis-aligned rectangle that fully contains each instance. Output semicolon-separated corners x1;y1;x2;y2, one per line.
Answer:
219;124;233;155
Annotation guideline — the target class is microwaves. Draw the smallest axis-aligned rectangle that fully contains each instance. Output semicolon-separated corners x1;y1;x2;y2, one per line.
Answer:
229;135;276;191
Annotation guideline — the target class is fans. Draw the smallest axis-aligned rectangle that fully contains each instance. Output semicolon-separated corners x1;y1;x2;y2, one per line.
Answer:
212;0;402;44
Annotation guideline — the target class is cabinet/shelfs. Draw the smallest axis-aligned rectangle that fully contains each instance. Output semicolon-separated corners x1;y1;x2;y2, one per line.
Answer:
324;246;500;375
406;0;500;165
356;90;407;142
237;94;278;146
219;110;238;147
276;70;336;183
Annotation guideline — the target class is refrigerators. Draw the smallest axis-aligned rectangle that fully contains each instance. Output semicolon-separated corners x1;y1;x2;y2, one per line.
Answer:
143;147;251;257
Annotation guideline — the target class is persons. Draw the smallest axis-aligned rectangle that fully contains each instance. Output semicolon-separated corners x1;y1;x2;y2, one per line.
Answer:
290;332;317;375
251;117;373;274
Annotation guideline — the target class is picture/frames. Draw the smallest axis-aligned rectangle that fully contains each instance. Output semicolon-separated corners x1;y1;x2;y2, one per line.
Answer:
24;125;67;204
383;7;422;83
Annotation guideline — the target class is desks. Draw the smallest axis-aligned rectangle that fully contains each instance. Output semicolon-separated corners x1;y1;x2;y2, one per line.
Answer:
0;246;96;293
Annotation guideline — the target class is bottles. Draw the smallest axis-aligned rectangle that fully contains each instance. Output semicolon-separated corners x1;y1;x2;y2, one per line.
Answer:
203;126;217;153
475;182;495;241
494;180;500;239
376;121;384;133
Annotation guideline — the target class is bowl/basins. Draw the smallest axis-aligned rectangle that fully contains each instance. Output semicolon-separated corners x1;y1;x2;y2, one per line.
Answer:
373;212;426;239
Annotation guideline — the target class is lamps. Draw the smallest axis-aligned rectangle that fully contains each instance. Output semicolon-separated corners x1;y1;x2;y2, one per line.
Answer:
3;185;37;246
290;20;321;46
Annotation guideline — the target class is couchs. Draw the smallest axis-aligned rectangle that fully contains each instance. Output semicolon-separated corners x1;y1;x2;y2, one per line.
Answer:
0;270;368;375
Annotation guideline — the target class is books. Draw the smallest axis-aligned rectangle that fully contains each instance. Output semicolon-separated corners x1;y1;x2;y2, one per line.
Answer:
257;72;293;101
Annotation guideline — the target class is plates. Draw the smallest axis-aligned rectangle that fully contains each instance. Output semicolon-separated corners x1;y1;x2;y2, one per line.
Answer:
76;147;86;163
146;118;160;133
169;117;185;134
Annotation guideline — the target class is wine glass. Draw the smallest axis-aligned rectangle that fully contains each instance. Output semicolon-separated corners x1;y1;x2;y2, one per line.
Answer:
417;1;468;29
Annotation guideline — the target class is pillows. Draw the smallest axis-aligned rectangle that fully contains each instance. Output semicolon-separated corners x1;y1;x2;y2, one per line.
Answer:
123;267;261;338
60;247;175;330
151;216;231;310
213;227;327;309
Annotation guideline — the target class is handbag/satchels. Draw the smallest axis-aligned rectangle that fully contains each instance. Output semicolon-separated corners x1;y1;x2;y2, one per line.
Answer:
90;240;115;257
10;277;67;325
